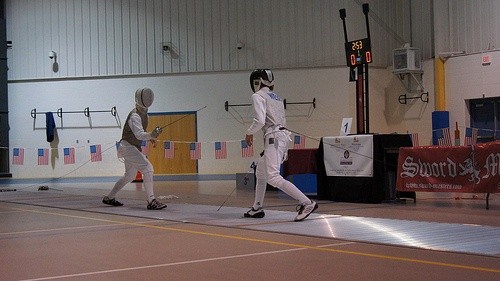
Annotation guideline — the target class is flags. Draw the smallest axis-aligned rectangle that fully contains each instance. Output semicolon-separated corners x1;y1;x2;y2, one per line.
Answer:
408;128;477;148
11;135;306;165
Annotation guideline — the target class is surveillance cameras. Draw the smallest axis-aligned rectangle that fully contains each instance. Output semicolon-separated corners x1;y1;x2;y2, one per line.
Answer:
237;43;243;49
49;52;54;59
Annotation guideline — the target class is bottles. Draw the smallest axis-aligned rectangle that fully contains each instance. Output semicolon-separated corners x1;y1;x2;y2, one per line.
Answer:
455;121;460;146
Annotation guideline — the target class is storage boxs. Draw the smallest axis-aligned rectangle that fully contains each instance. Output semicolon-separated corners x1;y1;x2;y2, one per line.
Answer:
236;173;254;189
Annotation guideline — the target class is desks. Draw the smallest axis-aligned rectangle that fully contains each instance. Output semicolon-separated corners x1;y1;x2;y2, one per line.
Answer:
316;134;417;205
383;141;500;210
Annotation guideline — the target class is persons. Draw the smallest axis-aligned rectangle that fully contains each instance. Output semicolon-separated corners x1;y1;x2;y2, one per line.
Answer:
243;69;318;222
102;89;168;209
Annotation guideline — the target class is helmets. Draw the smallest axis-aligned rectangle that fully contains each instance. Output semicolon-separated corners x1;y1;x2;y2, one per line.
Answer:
250;69;274;93
135;88;154;109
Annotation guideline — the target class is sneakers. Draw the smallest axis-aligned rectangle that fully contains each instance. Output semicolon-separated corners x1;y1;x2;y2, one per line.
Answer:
294;200;318;221
103;196;123;206
147;199;167;209
244;207;265;218
